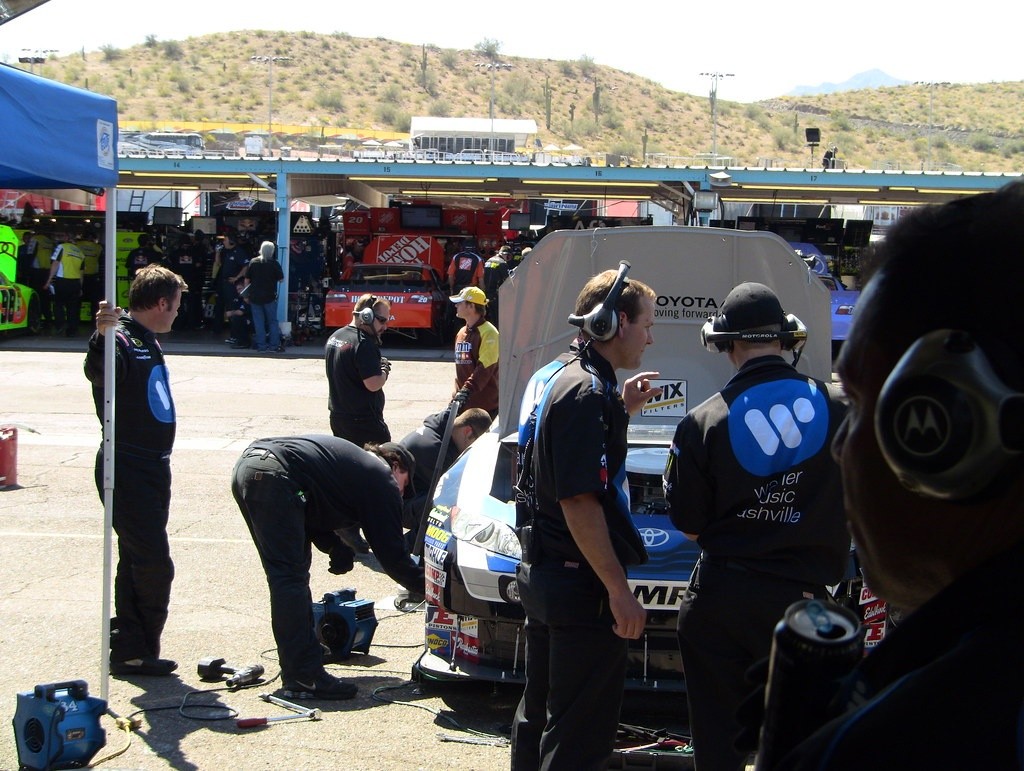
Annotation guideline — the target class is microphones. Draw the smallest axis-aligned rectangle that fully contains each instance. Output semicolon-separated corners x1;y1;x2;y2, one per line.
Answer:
369;323;383;346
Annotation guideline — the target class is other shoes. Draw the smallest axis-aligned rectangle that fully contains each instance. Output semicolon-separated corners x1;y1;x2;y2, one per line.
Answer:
110;658;178;675
259;349;267;353
269;346;285;352
225;338;236;343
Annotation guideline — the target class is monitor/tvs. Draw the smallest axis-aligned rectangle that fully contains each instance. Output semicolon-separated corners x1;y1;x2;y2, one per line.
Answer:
399;205;443;231
509;213;531;230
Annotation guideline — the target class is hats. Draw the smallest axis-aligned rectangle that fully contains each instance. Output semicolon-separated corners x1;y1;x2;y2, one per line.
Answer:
379;442;417;499
449;286;489;305
138;234;150;241
713;281;785;331
466;242;476;253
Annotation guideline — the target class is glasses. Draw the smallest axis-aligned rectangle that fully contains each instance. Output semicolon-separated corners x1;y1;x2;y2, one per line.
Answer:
374;313;386;324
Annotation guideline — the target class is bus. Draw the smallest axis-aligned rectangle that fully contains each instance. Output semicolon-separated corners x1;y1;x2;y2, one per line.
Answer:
140;132;205;155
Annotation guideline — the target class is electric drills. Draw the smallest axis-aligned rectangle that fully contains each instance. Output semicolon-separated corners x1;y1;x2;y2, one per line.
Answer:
197;656;265;687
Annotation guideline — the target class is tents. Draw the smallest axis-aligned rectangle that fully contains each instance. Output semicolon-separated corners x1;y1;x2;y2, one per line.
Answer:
0;61;121;706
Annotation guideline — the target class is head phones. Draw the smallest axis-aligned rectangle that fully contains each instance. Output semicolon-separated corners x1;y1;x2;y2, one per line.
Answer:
229;233;234;245
352;295;378;325
700;314;807;353
870;196;1024;506
569;259;631;342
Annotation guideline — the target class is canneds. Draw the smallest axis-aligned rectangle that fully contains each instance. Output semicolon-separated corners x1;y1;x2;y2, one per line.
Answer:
754;599;865;771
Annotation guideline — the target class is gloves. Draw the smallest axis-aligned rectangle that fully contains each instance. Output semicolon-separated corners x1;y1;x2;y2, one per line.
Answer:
419;567;426;594
328;542;355;575
381;357;391;378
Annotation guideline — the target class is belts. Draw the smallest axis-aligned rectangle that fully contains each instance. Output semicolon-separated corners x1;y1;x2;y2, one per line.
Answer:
251;448;280;460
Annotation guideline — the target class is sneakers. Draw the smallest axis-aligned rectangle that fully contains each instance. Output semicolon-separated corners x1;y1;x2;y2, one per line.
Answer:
282;671;358;700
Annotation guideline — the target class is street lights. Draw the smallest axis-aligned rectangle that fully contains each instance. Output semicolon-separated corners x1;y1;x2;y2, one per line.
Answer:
700;72;736;167
475;63;513;164
252;56;293;156
914;81;951;171
22;48;60;78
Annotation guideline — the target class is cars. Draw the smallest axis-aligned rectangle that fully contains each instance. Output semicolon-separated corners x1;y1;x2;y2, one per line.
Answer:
413;223;837;716
0;224;41;337
324;236;447;344
786;242;860;361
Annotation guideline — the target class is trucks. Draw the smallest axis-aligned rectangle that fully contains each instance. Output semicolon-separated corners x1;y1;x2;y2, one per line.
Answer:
244;136;264;156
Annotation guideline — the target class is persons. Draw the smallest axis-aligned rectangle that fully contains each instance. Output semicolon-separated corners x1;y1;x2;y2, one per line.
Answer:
0;222;847;771
752;175;1024;771
